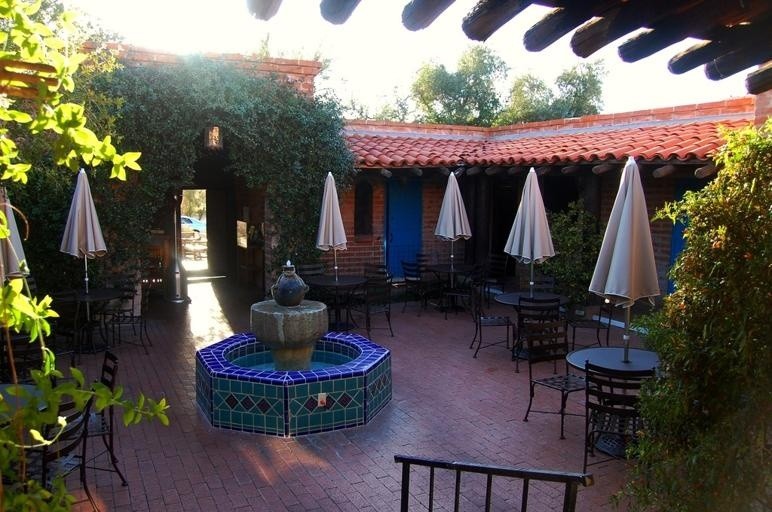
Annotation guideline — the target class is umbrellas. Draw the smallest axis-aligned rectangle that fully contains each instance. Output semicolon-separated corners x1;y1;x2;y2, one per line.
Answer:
60;168;108;320
589;156;660;434
315;171;347;321
503;167;556;351
434;169;472;305
0;179;30;364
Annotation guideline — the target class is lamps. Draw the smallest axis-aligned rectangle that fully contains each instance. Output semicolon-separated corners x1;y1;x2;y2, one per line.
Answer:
203;126;224;151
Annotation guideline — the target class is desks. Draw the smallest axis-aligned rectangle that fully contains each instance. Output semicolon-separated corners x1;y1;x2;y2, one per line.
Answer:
567;347;665;432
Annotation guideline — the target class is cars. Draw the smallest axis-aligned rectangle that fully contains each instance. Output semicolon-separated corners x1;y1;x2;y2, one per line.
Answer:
180;216;207;234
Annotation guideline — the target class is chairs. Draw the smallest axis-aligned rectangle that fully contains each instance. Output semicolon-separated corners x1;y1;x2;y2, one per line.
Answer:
392;452;595;512
466;276;616;374
523;322;599;441
293;251;503;340
1;256;155;512
583;359;659;490
